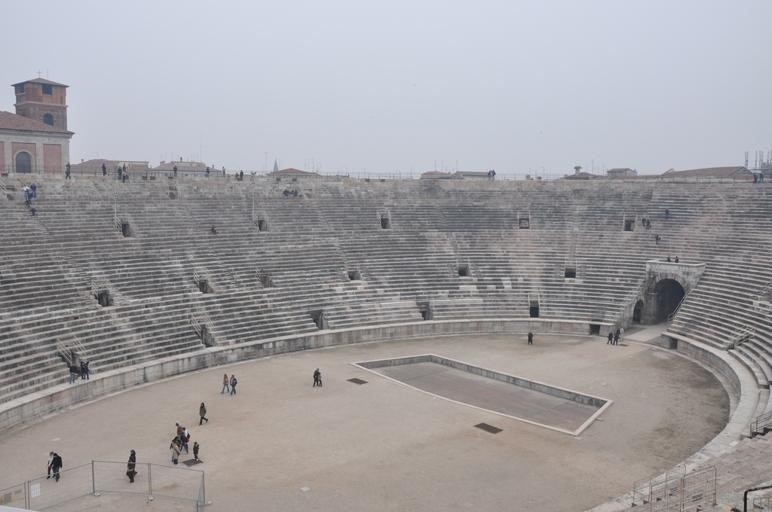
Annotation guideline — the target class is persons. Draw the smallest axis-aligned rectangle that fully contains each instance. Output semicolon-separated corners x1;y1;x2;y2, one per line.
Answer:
65;162;71;179
752;174;757;183
83;360;90;379
674;256;679;262
70;364;76;384
317;372;323;387
80;361;86;379
126;448;138;483
171;422;200;465
46;450;55;479
173;165;178;177
606;326;625;346
199;402;208;425
222;166;226;177
31;184;37;198
240;169;244;181
100;161;130;181
229;374;238;395
527;331;533;345
313;368;319;386
757;173;764;183
52;452;63;482
20;183;34;200
220;373;230;393
